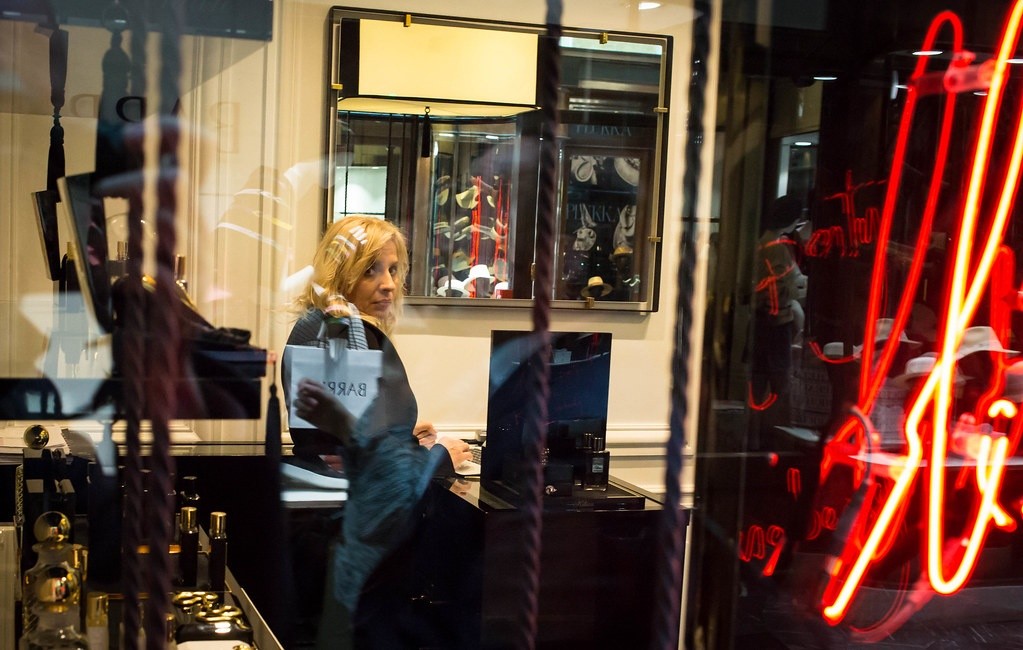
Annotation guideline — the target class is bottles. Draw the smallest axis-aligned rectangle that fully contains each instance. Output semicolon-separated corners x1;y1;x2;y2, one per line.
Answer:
581;437;609;488
210;511;227;602
179;506;199;586
582;433;594;453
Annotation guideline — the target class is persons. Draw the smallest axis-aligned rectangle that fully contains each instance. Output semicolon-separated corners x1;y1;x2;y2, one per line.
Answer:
291;325;450;650
825;319;1023;459
281;216;474;477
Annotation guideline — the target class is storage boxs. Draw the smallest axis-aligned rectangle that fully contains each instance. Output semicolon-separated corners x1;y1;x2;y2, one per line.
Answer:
186;341;267;382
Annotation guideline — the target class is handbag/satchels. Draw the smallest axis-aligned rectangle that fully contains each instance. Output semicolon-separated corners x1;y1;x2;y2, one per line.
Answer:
284;301;384;429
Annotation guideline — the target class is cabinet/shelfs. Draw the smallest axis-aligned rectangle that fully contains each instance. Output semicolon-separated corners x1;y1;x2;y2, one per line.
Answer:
681;1;1023;650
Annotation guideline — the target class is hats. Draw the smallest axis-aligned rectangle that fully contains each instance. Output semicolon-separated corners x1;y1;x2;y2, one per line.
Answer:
873;318;923;350
956;326;1021;361
437;280;470;298
815;341;862;360
463;265;495;291
610;246;633;263
580;275;613;299
1002;361;1023;403
894;357;964;382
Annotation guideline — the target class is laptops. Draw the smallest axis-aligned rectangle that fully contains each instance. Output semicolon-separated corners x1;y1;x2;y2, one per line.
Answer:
454;441;487;476
